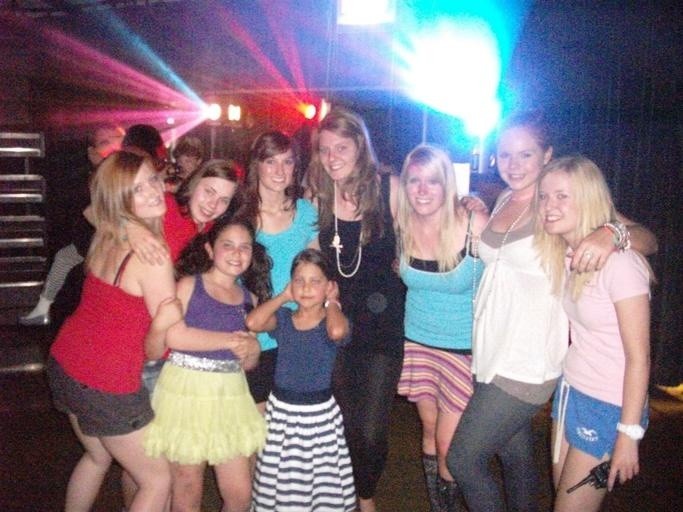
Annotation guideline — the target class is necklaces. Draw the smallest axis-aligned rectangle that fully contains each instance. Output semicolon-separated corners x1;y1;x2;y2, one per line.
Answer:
472;195;534;320
330;181;362;277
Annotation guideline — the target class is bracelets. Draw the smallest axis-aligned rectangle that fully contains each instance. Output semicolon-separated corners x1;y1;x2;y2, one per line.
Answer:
598;223;631;250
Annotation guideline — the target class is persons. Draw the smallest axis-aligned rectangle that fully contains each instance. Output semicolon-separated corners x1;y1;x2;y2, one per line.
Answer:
46;150;170;512
172;136;203;178
83;160;241;271
301;110;487;511
142;217;272;512
536;154;652;511
233;131;320;415
18;125;166;329
445;110;660;512
394;145;489;511
246;248;355;511
50;126;125;257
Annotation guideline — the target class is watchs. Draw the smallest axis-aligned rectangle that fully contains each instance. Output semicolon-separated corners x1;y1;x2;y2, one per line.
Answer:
324;300;342;309
617;423;645;441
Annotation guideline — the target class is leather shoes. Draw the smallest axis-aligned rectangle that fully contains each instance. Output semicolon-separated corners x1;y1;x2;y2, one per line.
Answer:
16;315;50;327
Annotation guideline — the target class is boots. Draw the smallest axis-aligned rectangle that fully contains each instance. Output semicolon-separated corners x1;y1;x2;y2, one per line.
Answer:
439;473;465;512
422;452;446;512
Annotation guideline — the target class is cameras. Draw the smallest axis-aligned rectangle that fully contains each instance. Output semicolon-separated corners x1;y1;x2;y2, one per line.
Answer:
166;166;182;176
